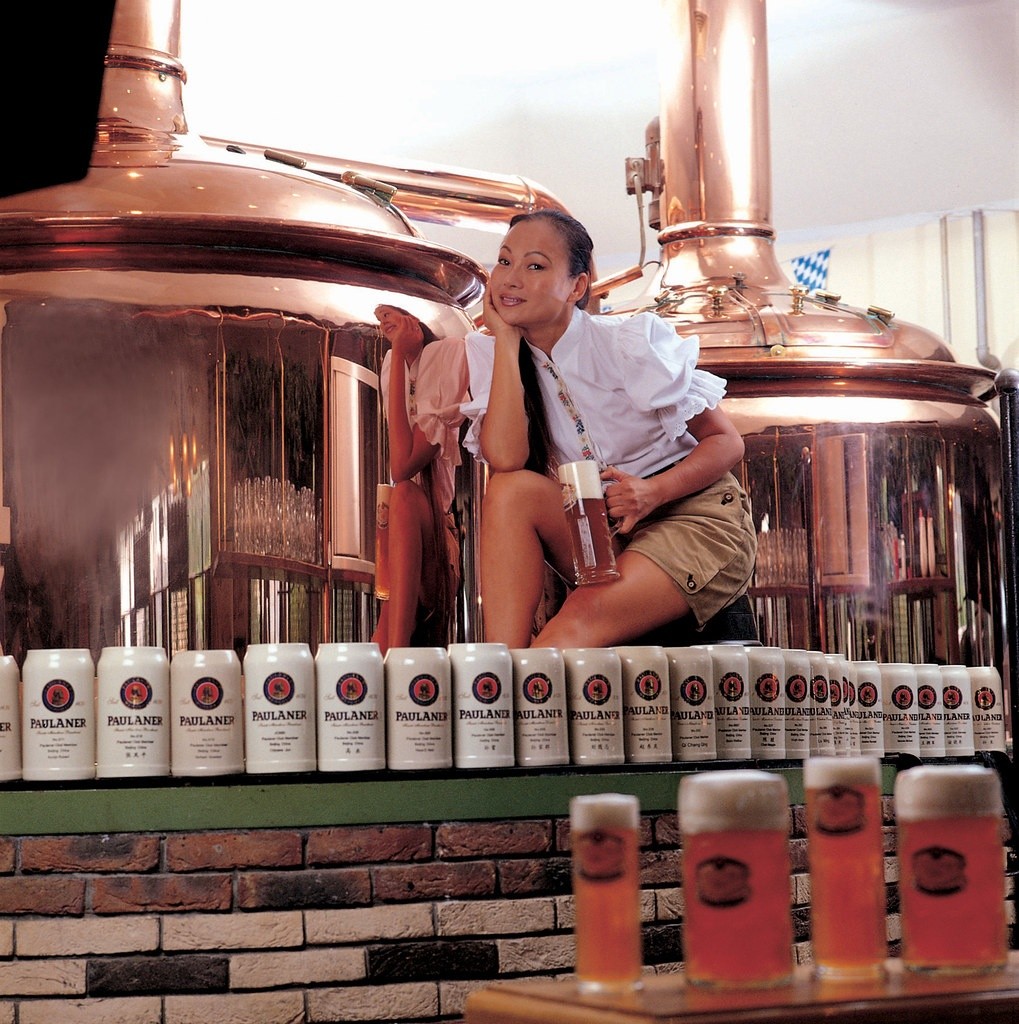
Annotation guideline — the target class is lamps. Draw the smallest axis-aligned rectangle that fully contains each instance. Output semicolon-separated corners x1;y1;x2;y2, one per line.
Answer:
753;449;809;585
169;363;198;500
233;329;317;564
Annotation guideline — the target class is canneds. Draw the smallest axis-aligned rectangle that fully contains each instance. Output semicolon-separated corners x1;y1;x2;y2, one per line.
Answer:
0;640;1007;782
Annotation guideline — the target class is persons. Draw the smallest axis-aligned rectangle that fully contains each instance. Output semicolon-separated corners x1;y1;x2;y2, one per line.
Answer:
460;210;758;647
373;304;472;655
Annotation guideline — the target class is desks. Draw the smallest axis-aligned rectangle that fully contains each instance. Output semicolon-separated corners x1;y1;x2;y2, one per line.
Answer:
466;950;1019;1024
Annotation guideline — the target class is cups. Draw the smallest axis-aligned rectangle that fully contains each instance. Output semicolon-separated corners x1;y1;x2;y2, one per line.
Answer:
677;769;797;992
891;767;1011;978
566;791;643;996
373;482;391;602
557;459;624;585
804;755;889;986
